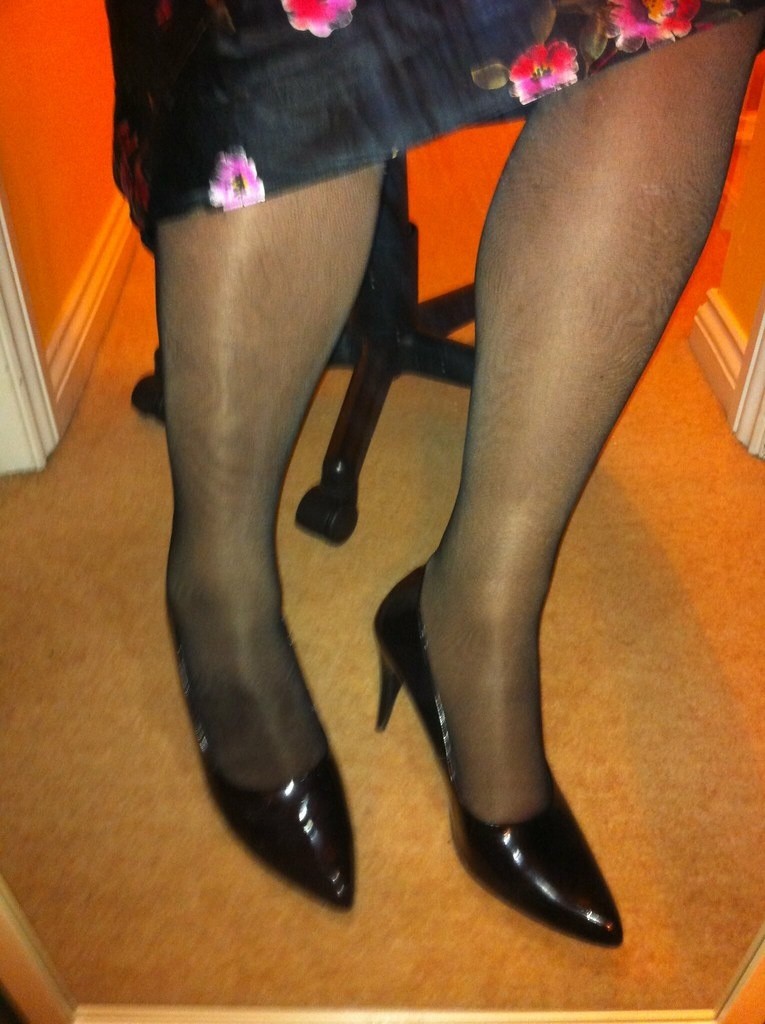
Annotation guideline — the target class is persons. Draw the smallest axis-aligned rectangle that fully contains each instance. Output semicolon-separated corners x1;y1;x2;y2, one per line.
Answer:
104;0;765;950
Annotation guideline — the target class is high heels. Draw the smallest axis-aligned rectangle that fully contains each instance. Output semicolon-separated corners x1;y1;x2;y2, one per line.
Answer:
201;728;358;912
374;566;624;948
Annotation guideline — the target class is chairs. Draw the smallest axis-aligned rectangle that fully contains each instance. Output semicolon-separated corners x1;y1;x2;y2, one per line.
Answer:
129;154;481;546
